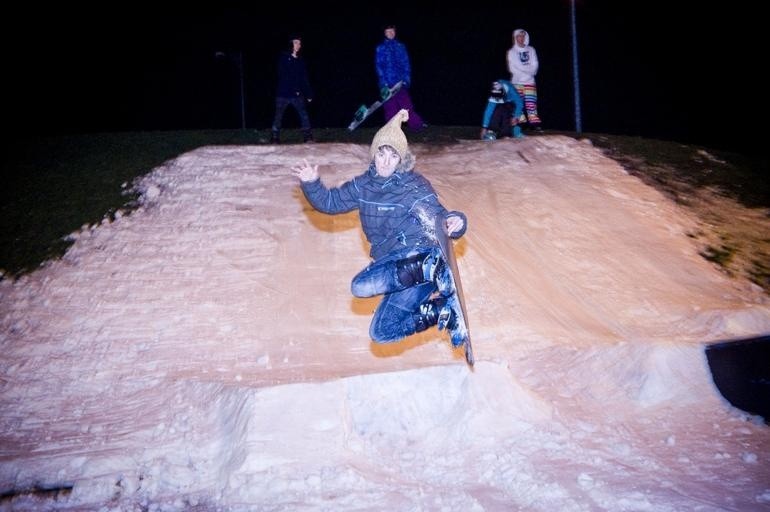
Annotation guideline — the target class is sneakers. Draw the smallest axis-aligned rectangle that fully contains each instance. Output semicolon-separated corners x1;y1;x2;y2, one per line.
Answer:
518;122;546;132
483;129;497;140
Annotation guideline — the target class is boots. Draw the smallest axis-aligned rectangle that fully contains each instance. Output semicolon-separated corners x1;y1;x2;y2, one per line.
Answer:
304;127;313;144
271;129;280;145
414;287;468;348
397;247;455;298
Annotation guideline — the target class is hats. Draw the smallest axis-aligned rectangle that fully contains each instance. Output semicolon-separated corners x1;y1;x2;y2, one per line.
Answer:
369;108;410;163
489;81;504;98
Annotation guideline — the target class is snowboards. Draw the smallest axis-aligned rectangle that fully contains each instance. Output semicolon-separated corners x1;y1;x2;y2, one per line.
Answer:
434;212;474;372
348;81;402;132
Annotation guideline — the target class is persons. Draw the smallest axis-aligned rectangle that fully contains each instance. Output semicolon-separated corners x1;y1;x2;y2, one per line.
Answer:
269;33;313;144
505;28;544;133
373;23;431;135
479;78;527;141
290;107;468;350
